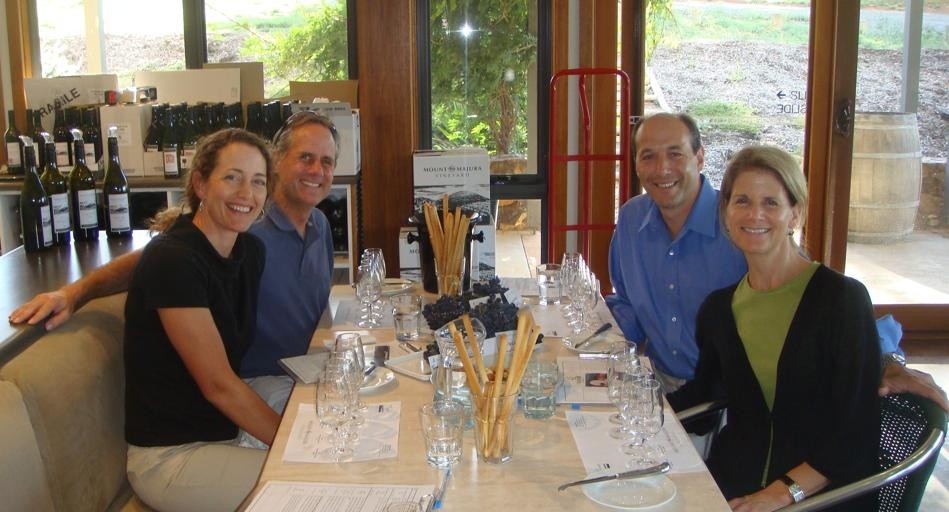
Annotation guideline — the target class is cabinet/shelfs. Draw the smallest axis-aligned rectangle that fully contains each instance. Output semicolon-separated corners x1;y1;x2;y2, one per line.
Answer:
1;180;356;287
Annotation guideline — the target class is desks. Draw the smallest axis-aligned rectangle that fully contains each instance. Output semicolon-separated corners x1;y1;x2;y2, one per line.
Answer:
0;224;164;357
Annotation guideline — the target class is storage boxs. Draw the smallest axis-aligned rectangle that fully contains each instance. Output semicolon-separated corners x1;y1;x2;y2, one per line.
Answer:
16;55;362;179
398;147;498;283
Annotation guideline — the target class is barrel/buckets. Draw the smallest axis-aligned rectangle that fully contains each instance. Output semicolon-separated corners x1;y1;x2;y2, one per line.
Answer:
846;110;922;245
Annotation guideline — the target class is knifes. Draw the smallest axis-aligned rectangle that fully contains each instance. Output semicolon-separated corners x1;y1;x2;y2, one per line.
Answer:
575;322;613;348
559;463;670;491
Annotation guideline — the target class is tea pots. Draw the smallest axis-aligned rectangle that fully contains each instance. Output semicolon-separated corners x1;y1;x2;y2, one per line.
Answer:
432;318;488;430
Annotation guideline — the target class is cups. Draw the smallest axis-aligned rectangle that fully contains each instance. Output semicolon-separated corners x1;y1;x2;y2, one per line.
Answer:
390;294;421;342
419;344;558;468
536;264;563;305
435;256;466;300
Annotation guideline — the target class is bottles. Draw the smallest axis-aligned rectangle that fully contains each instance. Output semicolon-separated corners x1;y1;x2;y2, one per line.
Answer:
3;98;132;253
143;98;294;179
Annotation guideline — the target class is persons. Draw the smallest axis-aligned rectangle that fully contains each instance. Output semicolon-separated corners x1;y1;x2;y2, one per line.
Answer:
663;146;882;512
601;110;949;463
122;129;295;512
7;107;341;413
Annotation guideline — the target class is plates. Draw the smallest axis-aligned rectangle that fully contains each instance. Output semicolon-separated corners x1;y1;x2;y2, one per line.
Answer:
382;278;415;295
581;467;678;511
560;332;619;357
359;350;431;394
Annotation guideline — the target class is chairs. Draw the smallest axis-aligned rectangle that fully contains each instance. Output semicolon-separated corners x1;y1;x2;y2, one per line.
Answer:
678;383;949;510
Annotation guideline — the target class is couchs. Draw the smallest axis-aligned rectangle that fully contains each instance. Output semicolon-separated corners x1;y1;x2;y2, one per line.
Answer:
0;289;156;512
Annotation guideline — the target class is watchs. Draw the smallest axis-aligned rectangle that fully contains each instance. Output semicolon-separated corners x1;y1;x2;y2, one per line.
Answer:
881;351;905;373
778;474;805;503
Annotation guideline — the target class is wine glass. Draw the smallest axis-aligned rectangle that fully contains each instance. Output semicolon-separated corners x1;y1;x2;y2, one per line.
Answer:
606;340;664;470
316;332;366;461
559;251;601;337
356;248;387;329
